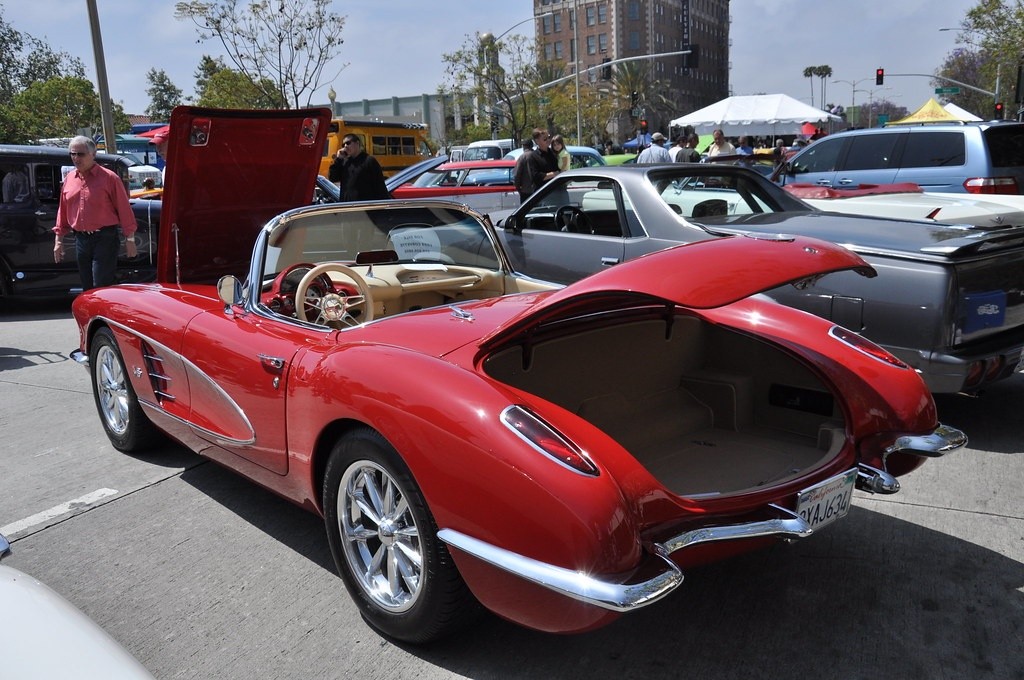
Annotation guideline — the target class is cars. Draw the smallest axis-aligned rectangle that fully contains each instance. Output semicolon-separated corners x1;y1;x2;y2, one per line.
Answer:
388;161;1024;403
582;154;1024;228
0;144;162;308
311;138;610;216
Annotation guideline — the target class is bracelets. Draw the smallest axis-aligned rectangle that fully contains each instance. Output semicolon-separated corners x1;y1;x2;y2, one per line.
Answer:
126;237;134;242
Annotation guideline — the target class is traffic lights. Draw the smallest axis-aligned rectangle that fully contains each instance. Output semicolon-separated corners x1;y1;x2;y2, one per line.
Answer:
639;120;648;135
876;69;884;85
994;103;1004;119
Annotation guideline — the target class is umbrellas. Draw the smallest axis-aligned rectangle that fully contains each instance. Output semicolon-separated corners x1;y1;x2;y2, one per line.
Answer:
883;98;966;124
944;102;983;122
802;124;817;135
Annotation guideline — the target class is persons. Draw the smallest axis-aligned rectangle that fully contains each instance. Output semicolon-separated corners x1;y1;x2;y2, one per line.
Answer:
637;132;672;194
754;128;826;173
3;164;31;202
551;134;570;189
668;136;688;163
54;136;137;292
513;139;536;204
532;128;569;205
329;134;388;203
735;136;754;169
606;140;625;155
149;127;170;187
676;133;701;163
143;178;154;191
708;129;738;185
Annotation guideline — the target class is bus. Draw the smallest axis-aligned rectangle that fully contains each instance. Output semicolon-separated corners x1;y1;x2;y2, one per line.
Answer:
317;120;439;182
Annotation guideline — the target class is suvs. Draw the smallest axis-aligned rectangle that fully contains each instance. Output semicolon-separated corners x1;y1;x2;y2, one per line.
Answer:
62;151;162;187
763;121;1024;194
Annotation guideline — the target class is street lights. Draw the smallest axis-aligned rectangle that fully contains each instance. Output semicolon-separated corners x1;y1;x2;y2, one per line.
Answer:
328;86;337;119
488;13;553;139
939;28;1002;103
832;76;905;129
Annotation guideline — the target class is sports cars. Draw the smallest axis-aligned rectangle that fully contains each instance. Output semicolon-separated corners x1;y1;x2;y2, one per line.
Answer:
68;104;967;636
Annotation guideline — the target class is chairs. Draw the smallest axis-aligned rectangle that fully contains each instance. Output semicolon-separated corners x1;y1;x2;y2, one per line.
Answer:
666;199;728;218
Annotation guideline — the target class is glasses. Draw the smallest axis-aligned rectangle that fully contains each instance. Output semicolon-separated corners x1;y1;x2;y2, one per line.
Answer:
68;151;88;157
543;135;551;141
341;139;352;148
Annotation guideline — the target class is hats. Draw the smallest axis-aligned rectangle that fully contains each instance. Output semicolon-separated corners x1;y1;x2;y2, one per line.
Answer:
519;139;534;147
650;132;667;141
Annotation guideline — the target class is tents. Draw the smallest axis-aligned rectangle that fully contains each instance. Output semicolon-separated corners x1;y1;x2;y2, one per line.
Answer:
623;133;672;150
668;93;842;146
